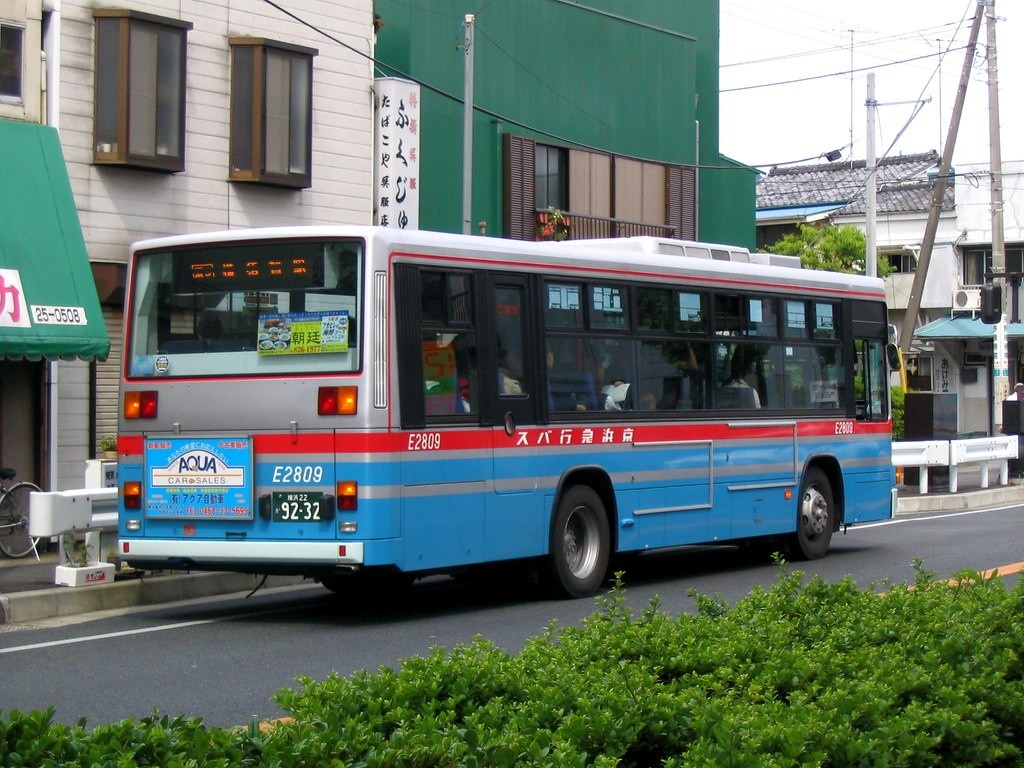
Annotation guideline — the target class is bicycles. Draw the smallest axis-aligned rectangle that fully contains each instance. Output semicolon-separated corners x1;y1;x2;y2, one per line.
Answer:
0;467;44;562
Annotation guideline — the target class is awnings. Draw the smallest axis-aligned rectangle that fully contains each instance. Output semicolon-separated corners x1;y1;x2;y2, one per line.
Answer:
0;115;109;366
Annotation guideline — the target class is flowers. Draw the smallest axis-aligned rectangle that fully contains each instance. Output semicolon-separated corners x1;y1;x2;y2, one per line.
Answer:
534;206;569;240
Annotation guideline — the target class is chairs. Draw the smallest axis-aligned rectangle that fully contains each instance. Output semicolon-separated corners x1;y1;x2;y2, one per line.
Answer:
452;369;793;414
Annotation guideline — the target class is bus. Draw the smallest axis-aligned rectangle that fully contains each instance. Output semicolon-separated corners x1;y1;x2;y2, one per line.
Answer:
116;224;901;596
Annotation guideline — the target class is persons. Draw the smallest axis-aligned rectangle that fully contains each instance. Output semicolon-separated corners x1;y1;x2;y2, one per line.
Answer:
226;315;257;350
426;305;787;419
344;317;357;348
196;314;223;343
